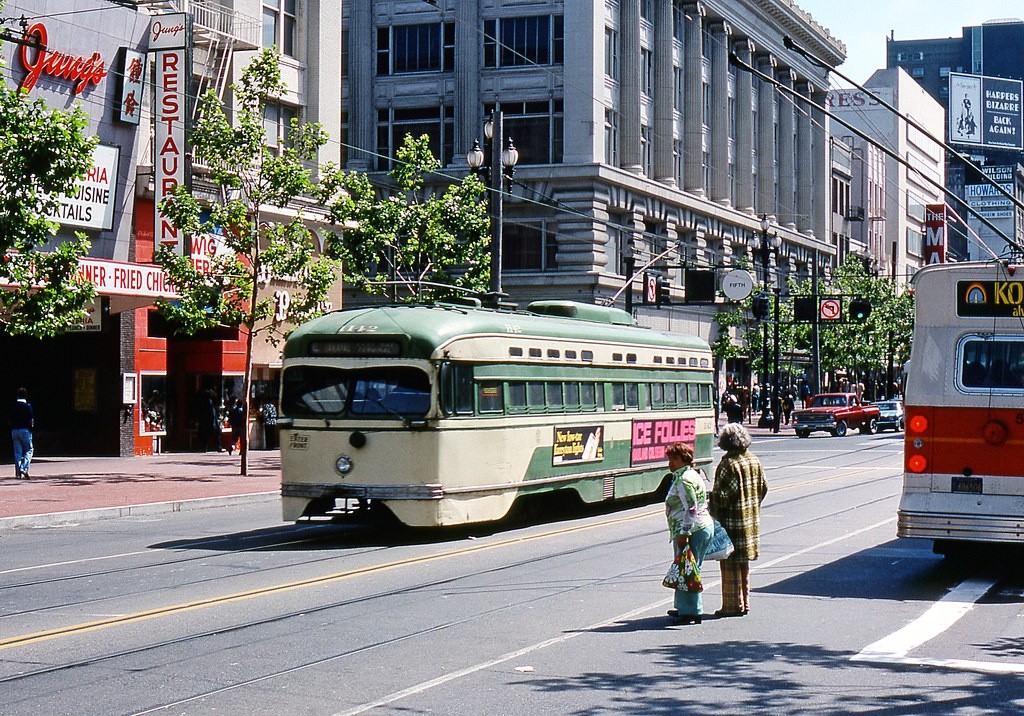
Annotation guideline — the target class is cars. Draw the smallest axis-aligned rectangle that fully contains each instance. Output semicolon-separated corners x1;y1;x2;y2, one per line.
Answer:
869;397;904;433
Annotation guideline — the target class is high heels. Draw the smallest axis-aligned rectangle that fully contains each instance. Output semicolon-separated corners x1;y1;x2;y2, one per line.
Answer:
667;610;678;617
666;613;702;625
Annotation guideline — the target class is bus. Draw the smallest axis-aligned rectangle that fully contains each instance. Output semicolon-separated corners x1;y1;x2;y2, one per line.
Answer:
727;36;1022;568
274;242;718;539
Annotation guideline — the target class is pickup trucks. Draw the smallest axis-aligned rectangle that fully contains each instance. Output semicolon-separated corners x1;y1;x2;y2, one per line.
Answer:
791;392;882;438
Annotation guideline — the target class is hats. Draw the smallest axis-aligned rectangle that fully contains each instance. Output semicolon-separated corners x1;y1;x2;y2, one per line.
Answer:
731;395;737;403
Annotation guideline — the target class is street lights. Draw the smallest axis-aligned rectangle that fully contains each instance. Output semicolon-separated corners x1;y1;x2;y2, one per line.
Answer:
749;211;775;429
466;108;519;253
859;245;881;402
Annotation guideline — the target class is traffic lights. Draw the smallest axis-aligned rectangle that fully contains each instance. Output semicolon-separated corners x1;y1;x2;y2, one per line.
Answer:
848;297;872;322
656;275;671;311
684;269;715;305
752;294;770;319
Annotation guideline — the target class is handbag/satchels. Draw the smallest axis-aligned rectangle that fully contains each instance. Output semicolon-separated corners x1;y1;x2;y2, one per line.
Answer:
701;517;735;560
661;545;703;593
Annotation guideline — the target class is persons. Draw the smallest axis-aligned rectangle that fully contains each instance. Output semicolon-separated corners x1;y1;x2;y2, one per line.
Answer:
202;393;243;455
260;396;277;450
841;378;902;401
722;382;810;426
709;423;768;616
11;388;34;479
666;443;715;620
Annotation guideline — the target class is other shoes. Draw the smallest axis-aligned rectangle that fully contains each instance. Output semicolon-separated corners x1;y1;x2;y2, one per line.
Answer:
20;469;31;480
229;446;233;455
744;611;747;615
16;475;20;479
716;610;743;617
